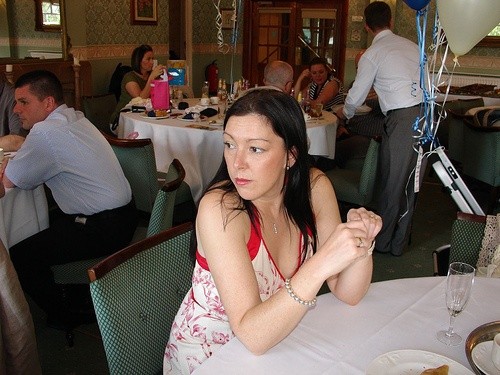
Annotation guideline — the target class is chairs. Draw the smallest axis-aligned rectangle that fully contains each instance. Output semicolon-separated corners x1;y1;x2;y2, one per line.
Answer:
49;94;500;375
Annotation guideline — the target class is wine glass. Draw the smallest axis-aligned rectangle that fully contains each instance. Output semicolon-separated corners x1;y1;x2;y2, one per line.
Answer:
436;262;476;346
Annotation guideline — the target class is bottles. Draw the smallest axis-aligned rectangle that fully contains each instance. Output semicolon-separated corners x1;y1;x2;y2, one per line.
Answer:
218;80;228;108
203;81;209;100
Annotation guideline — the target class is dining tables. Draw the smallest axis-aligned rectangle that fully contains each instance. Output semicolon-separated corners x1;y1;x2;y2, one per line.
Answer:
189;276;500;375
0;151;50;257
116;97;338;207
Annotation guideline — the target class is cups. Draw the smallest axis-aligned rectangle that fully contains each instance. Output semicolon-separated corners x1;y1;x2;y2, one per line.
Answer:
0;148;4;161
297;93;303;106
492;334;500;369
305;114;311;120
316;101;324;119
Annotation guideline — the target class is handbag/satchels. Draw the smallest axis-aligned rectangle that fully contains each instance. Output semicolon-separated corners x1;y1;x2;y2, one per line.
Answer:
132;106;146;113
151;80;169;109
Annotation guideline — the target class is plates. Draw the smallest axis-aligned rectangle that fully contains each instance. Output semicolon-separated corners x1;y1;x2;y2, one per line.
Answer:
366;349;475;375
140;111;169;121
471;341;500;375
177;114;206;122
208;120;225;125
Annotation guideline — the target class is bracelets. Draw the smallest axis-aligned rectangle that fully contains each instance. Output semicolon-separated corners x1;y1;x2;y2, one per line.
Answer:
367;240;375;256
284;277;317;308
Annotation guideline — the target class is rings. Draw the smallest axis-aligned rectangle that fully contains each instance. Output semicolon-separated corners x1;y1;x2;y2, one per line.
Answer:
356;237;364;248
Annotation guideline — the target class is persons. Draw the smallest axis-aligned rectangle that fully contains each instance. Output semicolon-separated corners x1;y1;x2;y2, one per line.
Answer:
0;70;140;331
0;158;8;198
293;58;344;112
163;89;382;375
262;61;294;93
109;45;164;135
338;48;385;151
337;1;431;256
0;73;24;136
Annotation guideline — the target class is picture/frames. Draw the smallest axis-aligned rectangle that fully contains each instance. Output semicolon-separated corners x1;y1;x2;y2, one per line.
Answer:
130;0;158;25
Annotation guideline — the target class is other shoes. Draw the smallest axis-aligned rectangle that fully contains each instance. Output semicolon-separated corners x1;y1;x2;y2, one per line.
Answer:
45;304;94;329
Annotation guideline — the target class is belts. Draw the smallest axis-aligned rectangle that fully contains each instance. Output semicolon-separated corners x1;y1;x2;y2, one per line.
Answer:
387;102;430;116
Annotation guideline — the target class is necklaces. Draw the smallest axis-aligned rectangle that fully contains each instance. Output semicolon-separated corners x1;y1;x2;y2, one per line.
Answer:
262;210;282;234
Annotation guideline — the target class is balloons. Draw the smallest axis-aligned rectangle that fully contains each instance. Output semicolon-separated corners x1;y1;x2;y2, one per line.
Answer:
402;0;432;12
437;0;500;61
